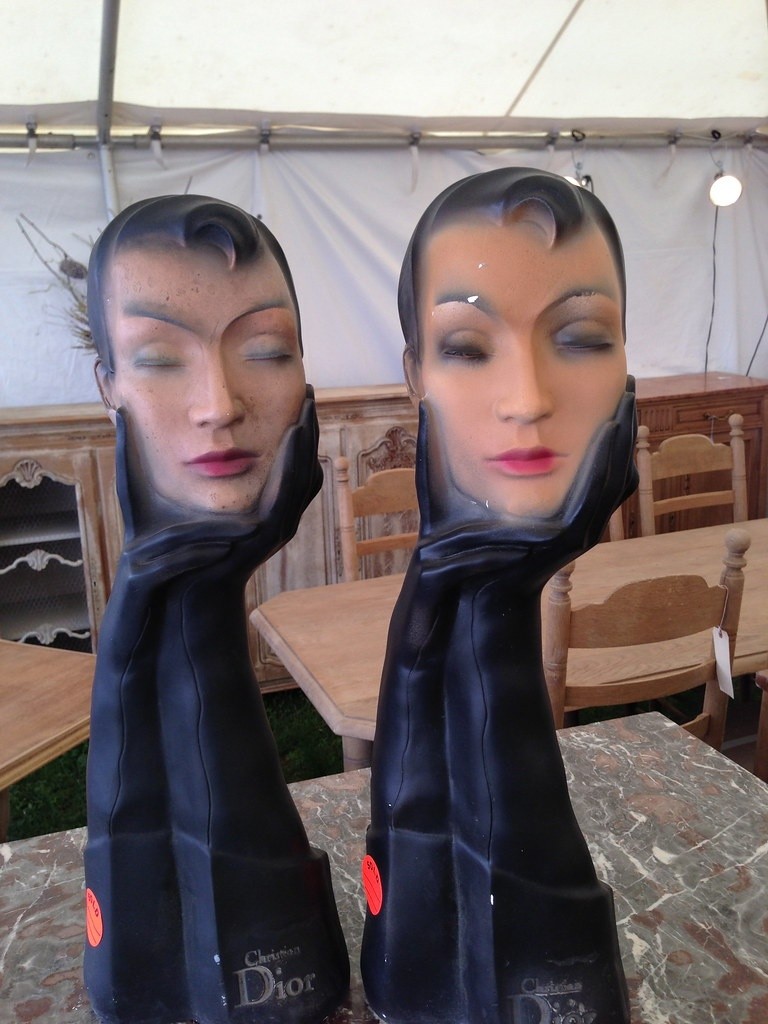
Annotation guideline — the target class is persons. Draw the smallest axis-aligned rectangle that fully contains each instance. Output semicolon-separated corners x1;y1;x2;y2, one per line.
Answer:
359;168;640;1024
82;191;352;1023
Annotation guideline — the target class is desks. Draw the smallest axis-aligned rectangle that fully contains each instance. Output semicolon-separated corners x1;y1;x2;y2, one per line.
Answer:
0;710;768;1024
0;639;97;841
249;518;767;774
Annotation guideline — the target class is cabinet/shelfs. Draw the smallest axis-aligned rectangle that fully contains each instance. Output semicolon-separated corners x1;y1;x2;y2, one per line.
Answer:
0;371;768;697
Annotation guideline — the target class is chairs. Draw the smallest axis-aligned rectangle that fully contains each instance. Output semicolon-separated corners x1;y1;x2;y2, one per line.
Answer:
334;457;421;582
636;415;748;536
544;530;752;753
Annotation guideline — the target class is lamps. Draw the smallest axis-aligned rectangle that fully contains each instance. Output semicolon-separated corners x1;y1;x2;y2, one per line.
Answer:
709;173;743;208
